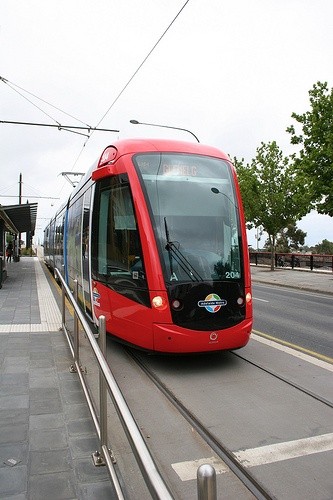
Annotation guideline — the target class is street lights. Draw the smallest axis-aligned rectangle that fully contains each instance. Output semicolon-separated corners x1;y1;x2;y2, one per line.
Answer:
129;120;200;143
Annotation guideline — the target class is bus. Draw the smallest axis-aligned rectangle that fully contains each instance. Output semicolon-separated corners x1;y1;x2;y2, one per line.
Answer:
42;136;256;360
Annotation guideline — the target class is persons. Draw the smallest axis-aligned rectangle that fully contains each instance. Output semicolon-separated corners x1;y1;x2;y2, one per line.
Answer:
74;227;87;260
5;241;12;263
